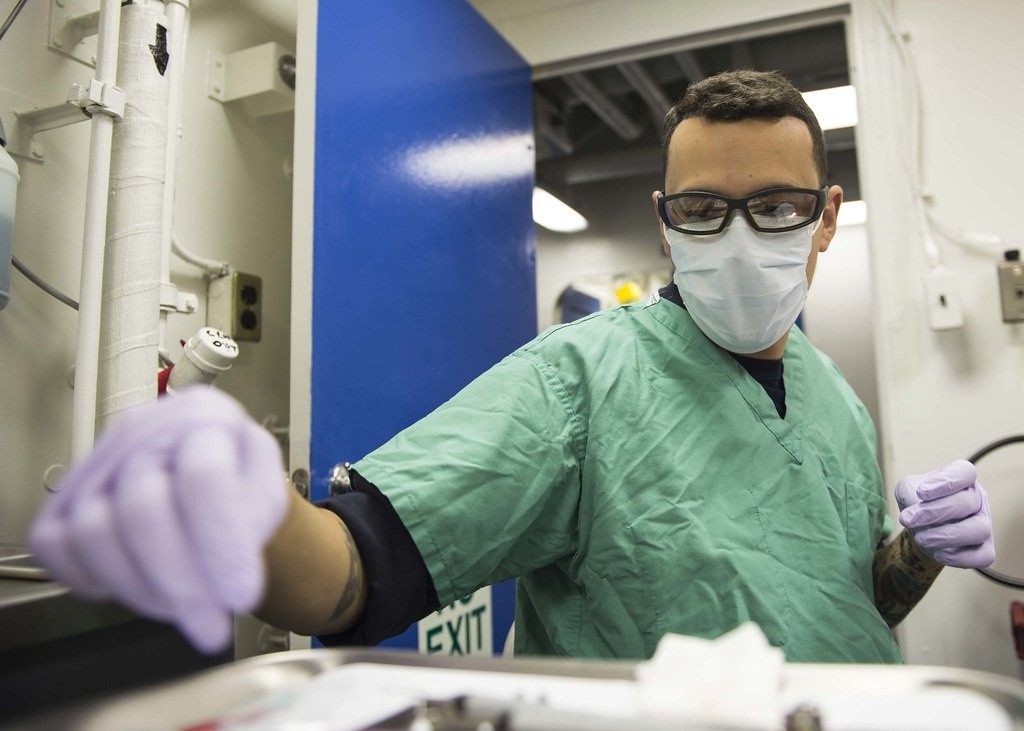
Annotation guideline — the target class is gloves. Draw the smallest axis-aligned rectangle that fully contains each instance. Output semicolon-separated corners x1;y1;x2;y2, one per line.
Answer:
892;458;996;569
19;380;291;654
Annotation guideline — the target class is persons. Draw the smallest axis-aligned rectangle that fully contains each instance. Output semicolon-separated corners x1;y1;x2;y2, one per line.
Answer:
25;67;999;666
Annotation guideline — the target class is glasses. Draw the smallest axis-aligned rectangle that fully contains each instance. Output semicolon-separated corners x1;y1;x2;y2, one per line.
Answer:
654;185;831;235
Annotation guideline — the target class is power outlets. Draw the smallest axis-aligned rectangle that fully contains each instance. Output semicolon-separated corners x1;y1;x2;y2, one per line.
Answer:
207;271;264;342
997;261;1024;323
